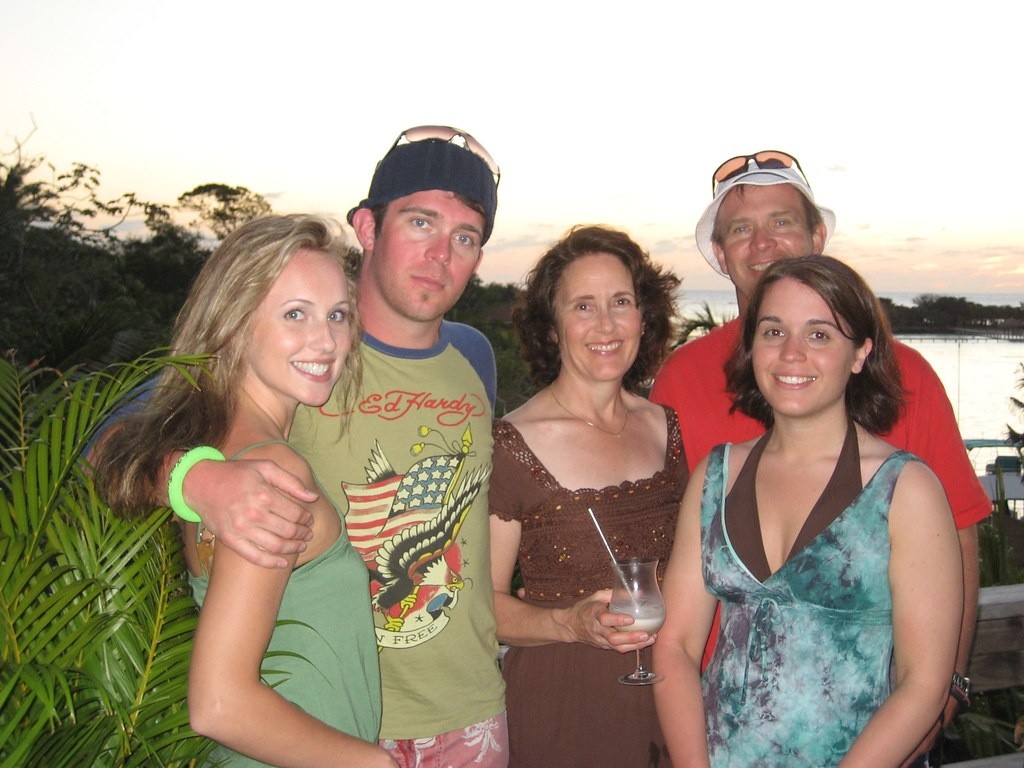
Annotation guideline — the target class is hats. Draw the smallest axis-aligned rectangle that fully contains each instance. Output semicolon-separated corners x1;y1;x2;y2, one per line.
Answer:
695;158;837;279
347;141;497;245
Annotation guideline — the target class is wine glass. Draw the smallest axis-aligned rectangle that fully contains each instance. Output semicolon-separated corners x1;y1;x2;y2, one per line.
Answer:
609;557;666;686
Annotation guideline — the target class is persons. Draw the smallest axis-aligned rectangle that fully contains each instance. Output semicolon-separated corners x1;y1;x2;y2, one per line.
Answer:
79;126;992;768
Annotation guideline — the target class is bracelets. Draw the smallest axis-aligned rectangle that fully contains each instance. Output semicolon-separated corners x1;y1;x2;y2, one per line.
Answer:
935;711;945;744
168;446;225;523
949;672;971;707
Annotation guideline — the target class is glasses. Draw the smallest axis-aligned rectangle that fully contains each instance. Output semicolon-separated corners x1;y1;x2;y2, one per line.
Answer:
382;125;500;186
712;150;811;200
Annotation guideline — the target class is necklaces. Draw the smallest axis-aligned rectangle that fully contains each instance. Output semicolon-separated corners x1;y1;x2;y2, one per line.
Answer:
550;382;628;439
